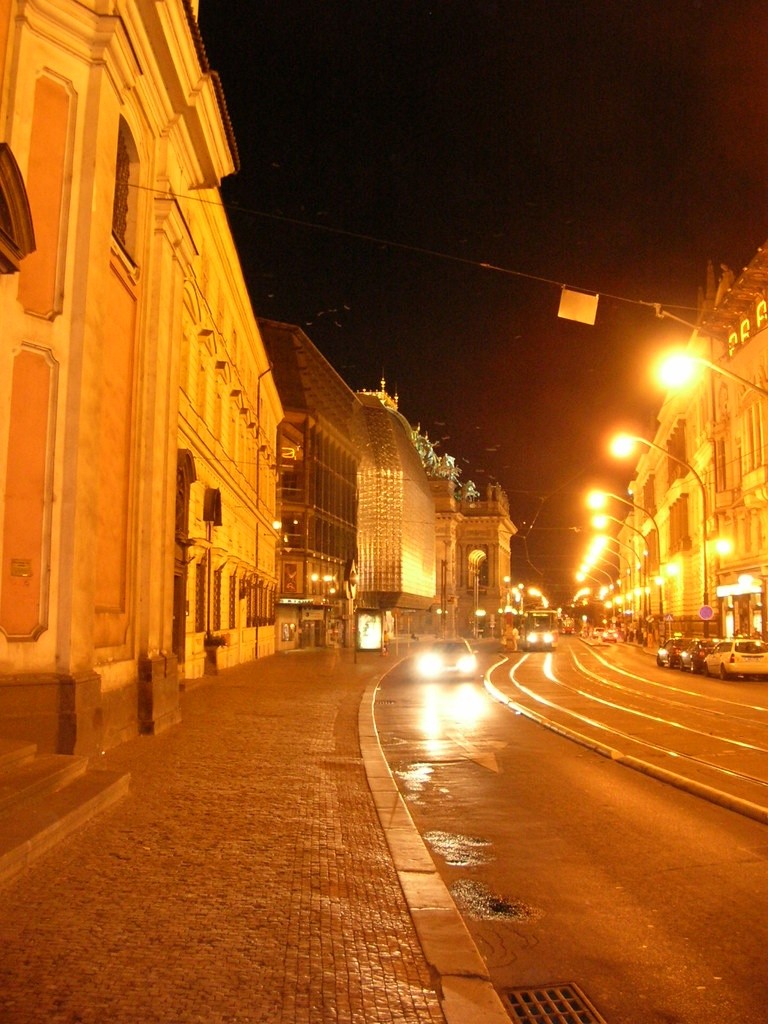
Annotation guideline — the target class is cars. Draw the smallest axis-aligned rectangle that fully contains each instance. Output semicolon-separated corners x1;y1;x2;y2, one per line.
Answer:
678;638;722;674
703;639;768;680
657;637;698;668
557;615;618;644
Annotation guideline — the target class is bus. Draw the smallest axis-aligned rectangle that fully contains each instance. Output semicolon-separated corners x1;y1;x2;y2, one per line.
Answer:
524;610;558;652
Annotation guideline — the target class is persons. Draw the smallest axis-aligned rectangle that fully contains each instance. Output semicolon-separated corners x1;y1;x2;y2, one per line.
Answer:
383;630;390;654
512;626;519;651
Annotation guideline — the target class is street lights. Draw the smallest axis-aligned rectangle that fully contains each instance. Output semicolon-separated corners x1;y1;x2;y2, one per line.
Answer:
590;512;653;615
610;433;713;640
576;533;643;645
583;490;664;621
